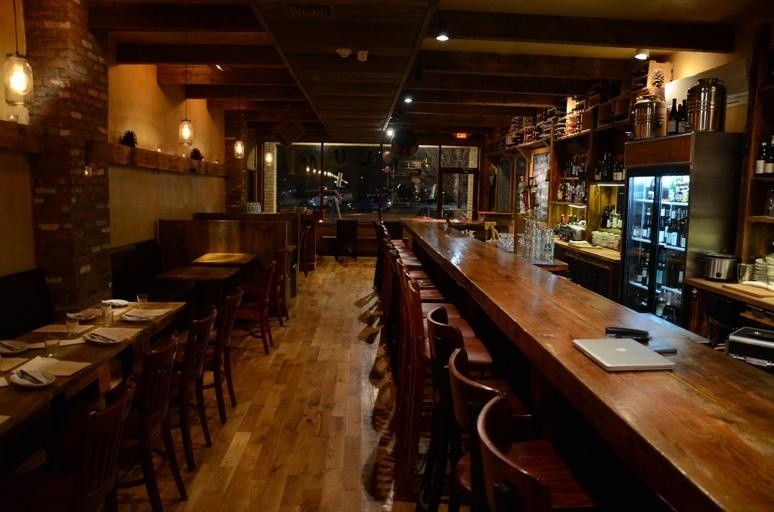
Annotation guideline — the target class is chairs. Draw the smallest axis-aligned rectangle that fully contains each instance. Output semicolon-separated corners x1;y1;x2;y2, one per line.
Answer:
331;219;358;262
239;250;288;328
118;335;188;509
370;215;602;510
205;286;245;426
235;264;275;356
4;379;137;509
134;306;219;472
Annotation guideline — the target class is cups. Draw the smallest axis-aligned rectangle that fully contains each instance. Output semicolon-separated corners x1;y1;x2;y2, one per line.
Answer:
497;232;514;252
100;301;112;325
524;226;555;264
591;230;620;250
136;294;147;313
246;201;261;213
737;254;773;283
64;319;78;338
43;335;60;358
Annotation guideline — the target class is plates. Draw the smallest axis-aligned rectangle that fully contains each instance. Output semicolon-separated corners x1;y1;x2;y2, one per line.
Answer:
83;332;123;344
119;315;152;321
10;371;55;387
0;339;28;354
100;298;129;307
65;312;94;321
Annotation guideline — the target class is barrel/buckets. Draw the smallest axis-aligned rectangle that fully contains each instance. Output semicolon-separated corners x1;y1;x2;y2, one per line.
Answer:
633;98;667;139
685;80;722;132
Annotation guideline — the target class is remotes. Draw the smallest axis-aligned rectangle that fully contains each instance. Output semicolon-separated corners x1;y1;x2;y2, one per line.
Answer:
606;326;648;337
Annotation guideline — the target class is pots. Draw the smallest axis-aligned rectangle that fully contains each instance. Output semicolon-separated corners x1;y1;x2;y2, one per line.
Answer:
703;247;739;282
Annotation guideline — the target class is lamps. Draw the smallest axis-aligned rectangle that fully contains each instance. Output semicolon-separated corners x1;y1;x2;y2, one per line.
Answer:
634;48;649;61
178;63;193;142
1;0;34;95
234;88;244;159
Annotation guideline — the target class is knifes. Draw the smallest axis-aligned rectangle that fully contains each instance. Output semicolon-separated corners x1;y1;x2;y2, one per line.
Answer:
92;332;117;341
20;369;45;383
0;341;16;351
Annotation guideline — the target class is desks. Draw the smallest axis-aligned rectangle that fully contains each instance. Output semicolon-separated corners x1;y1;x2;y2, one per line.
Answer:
190;250;258;304
399;216;774;512
2;301;187;433
158;266;240;320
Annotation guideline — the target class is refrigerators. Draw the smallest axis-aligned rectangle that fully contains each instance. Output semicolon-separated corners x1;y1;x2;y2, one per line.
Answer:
617;131;743;330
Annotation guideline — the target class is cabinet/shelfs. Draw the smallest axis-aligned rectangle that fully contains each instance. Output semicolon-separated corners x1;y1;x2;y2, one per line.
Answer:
735;43;774;271
547;119;630;252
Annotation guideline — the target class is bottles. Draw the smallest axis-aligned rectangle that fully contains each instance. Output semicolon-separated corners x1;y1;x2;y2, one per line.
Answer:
652;207;689;293
560;214;577;227
564;154;585;177
667;175;688;203
633;288;677;322
602;203;615;229
647;178;654;200
755;136;774;176
667;97;688;134
634;247;649;284
593;149;625;182
556;180;586;203
642;207;650;239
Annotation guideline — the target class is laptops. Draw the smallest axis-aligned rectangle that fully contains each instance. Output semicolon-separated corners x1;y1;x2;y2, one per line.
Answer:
572;338;676;372
